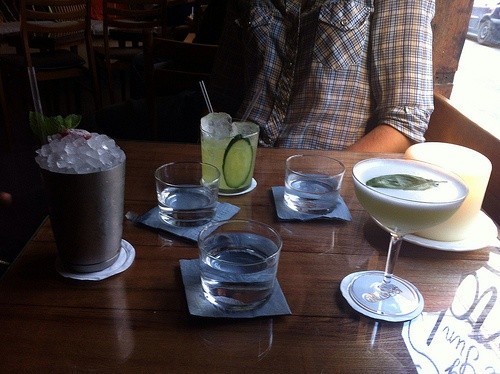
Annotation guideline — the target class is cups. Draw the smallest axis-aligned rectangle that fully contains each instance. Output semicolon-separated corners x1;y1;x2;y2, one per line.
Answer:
283;154;346;215
154;160;222;228
403;142;493;242
200;118;260;194
198;219;283;311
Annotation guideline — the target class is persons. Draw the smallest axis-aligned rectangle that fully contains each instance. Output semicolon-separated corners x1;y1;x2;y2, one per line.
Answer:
202;0;435;153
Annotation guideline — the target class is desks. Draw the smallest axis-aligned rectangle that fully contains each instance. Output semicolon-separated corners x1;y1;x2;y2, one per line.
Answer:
0;128;500;374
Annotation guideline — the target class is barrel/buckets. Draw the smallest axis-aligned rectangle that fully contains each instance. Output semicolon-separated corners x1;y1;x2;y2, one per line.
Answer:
35;155;127;273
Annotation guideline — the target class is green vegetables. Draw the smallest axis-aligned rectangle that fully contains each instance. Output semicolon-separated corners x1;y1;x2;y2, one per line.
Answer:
366;174;448;190
29;111;80;138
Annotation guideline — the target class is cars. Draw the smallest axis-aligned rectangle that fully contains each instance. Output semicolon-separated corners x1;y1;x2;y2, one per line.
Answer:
468;3;500;47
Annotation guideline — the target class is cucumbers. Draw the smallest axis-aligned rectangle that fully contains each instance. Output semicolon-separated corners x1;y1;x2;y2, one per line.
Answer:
222;134;253;189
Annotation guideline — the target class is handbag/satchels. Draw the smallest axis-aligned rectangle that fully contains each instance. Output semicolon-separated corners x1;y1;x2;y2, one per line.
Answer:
89;0;129;21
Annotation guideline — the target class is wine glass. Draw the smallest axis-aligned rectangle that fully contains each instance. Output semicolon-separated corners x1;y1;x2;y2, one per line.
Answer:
349;157;469;316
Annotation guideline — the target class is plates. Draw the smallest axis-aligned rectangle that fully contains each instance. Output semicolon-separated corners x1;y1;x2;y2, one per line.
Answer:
370;211;498;252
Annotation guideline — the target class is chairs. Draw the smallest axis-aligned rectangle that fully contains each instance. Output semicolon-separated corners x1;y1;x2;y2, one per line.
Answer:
0;0;219;150
